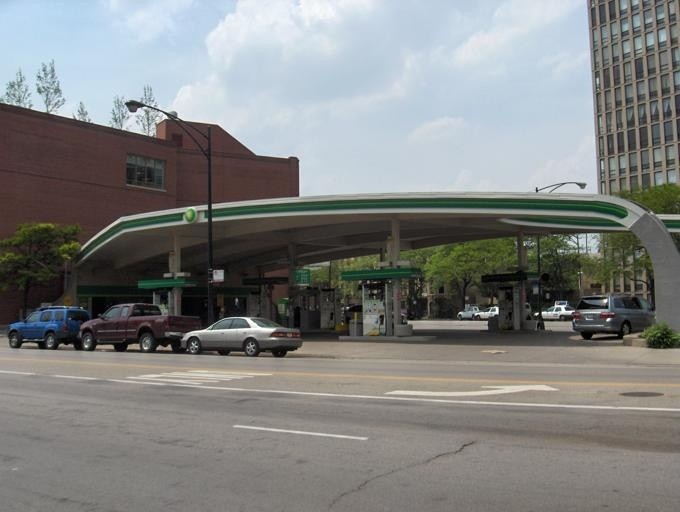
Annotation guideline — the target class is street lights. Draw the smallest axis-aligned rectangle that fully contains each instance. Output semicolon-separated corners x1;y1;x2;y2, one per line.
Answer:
535;181;587;304
124;98;215;327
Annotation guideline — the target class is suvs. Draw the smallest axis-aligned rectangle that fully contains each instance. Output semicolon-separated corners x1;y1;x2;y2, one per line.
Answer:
6;305;91;350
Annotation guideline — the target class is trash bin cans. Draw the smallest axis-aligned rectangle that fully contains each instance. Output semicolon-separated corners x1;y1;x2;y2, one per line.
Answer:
525;303;532;313
349;312;362;335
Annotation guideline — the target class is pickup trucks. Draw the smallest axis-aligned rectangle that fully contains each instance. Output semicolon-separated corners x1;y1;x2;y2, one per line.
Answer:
78;302;203;354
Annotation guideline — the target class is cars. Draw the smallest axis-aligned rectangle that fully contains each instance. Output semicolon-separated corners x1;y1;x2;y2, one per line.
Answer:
456;306;499;321
180;315;303;358
533;300;575;321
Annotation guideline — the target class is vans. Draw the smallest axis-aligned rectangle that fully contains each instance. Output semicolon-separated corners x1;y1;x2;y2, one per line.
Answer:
572;293;653;340
